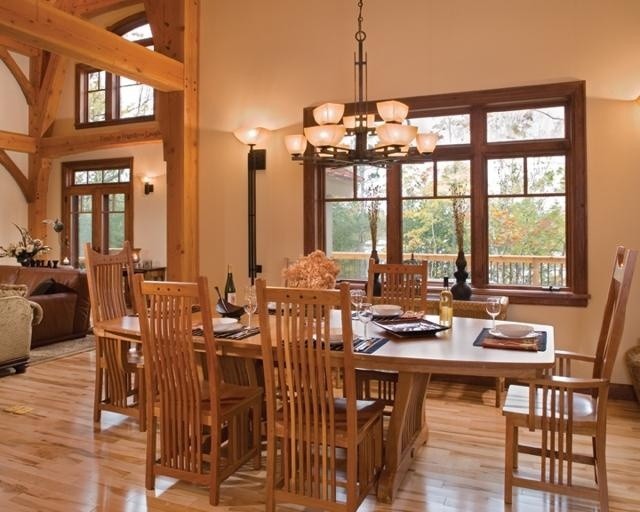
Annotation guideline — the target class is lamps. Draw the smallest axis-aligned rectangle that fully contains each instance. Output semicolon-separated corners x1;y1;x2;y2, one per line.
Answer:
233;127;265;284
282;0;439;171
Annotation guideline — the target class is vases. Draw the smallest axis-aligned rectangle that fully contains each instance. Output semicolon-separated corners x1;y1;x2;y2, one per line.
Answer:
364;251;381;297
450;251;470;298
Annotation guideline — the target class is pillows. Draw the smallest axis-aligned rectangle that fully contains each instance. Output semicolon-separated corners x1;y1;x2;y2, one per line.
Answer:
0;285;28;298
30;276;58;296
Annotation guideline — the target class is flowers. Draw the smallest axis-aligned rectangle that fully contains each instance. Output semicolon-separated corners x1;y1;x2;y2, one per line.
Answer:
0;218;53;263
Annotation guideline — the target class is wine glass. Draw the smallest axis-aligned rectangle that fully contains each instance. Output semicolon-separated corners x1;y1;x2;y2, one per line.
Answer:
355;303;373;342
349;288;365;320
485;296;502;330
243;286;258;330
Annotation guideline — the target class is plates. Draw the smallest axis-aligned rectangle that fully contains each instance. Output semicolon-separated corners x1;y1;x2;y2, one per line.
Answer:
373;309;404;318
489;324;541;339
197;317;242;334
213;300;247;319
372;318;447;338
311;327;358;346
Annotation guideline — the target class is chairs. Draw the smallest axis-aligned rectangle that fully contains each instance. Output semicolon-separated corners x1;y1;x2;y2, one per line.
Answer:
0;283;44;377
343;256;431;419
131;273;262;505
85;239;162;429
501;244;636;512
253;276;384;511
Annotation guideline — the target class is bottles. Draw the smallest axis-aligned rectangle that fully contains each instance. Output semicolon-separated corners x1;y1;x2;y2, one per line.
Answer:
438;275;453;329
224;263;237;307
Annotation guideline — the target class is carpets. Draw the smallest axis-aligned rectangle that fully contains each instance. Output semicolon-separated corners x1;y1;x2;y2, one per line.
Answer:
26;334;96;367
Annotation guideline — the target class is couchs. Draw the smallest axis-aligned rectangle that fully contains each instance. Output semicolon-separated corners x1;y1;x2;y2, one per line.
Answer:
0;264;91;350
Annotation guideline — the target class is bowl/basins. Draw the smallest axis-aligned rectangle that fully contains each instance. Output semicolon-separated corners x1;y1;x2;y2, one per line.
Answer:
496;323;534;337
370;304;401;313
208;316;238;329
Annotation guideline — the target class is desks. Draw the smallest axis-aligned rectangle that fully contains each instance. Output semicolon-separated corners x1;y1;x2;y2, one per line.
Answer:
122;265;166;307
334;287;508;409
